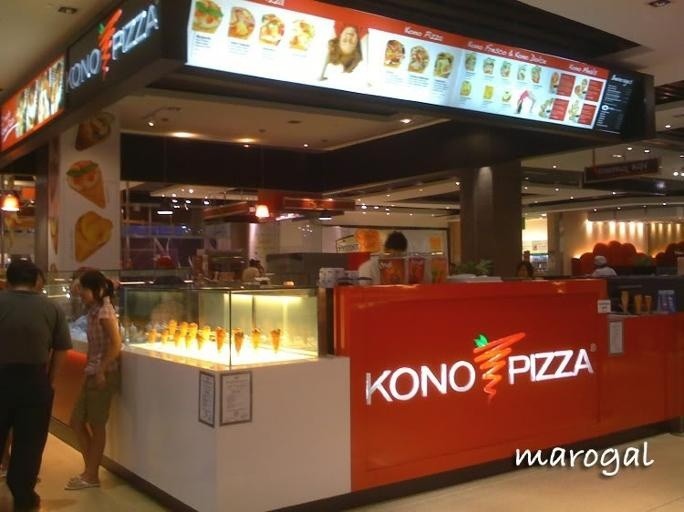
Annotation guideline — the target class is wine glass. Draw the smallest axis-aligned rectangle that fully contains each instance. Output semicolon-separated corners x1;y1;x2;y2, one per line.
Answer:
622;291;652;314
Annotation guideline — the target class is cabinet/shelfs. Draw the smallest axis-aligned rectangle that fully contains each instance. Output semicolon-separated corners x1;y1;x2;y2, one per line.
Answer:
119;284;327;371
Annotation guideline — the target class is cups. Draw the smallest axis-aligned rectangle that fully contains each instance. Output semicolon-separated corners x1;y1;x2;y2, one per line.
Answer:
319;267;358;289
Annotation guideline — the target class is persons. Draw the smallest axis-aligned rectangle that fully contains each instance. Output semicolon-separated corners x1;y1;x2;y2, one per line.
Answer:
0;268;54;479
242;258;260;282
357;231;408;284
256;260;264;273
326;21;368;73
516;260;534;279
0;259;73;512
64;269;124;490
592;254;617;277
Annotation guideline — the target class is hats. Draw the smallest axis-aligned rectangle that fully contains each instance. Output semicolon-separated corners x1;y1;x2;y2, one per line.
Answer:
594;255;607;265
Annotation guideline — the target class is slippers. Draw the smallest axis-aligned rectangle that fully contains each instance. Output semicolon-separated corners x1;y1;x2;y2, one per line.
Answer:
64;471;100;489
